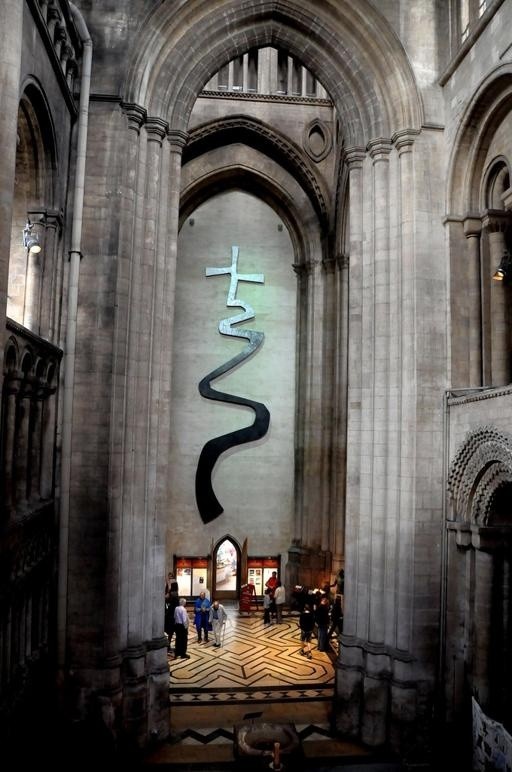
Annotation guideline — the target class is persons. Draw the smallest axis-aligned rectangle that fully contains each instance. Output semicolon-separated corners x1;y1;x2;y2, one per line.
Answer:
173;598;190;659
164;593;180;652
262;572;343;658
208;601;227;647
194;591;212;643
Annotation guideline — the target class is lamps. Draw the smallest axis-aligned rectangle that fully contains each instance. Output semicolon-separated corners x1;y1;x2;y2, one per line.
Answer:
493;252;512;283
21;221;43;256
